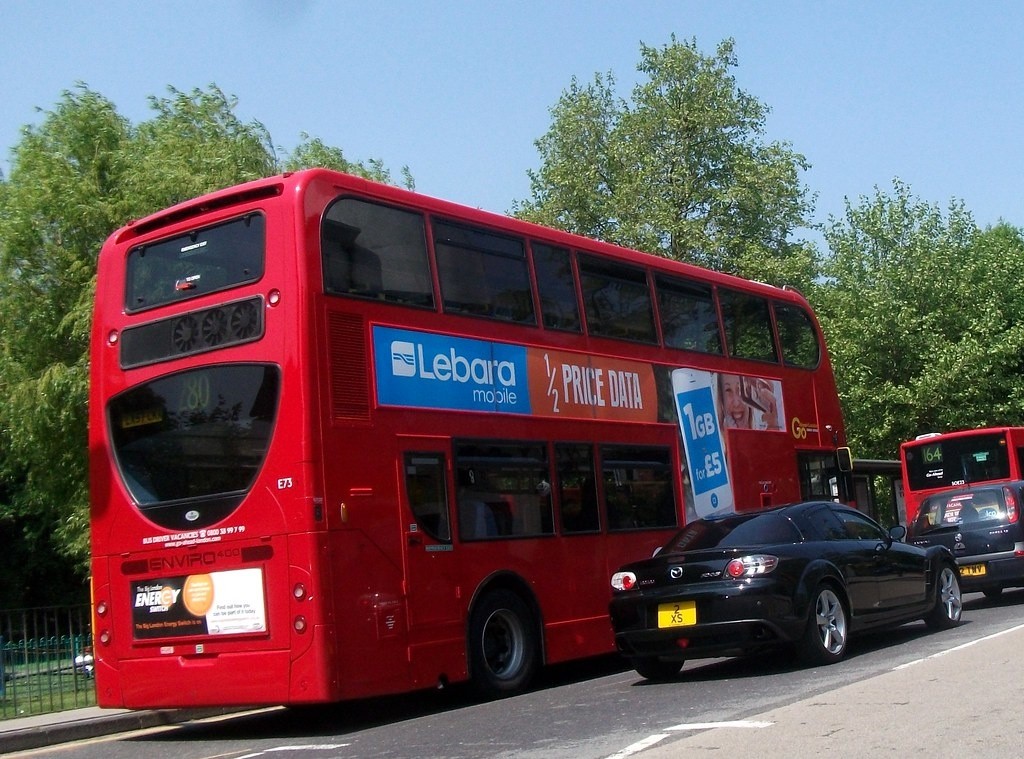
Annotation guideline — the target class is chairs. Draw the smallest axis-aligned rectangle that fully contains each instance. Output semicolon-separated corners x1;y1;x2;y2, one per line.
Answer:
496;493;542;534
440;502;485;540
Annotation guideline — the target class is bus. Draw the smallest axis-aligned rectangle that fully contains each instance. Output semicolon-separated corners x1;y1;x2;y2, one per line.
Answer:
89;167;856;721
900;428;1024;535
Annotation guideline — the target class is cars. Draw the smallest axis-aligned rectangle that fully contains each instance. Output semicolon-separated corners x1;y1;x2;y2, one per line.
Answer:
608;501;964;681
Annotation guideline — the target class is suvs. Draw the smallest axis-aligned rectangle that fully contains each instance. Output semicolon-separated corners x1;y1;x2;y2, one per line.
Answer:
906;479;1024;598
